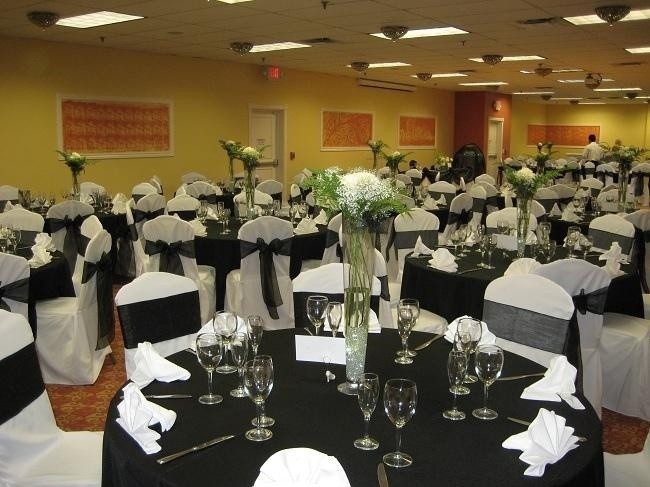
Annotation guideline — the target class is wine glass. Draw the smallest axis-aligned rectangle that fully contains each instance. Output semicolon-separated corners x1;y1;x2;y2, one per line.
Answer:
394;309;414;365
395;298;418;357
382;379;417;467
306;295;329;336
353;373;380;451
25;190;119;217
1;222;24;262
212;310;237;374
326;301;342;337
245;315;265;371
230;332;248;398
190;195;318;236
242;360;273;442
251;355;276;427
457;318;482;383
448;221;596;269
442;352;468;420
195;332;222;405
449;332;471;396
472;344;504;420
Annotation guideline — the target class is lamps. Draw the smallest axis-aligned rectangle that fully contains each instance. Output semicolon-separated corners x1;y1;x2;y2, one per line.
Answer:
27;12;60;32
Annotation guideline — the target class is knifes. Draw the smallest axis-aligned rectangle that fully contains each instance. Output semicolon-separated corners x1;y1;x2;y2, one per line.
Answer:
179;338;204;364
498;405;595;447
14;240;42;253
415;333;445;351
454;261;486;276
404;246;441;263
134;427;236;466
487;367;563;383
112;385;195;403
583;245;616;261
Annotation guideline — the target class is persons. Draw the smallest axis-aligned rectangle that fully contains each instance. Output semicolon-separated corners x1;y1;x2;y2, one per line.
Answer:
582;135;604;178
404;159;421;178
